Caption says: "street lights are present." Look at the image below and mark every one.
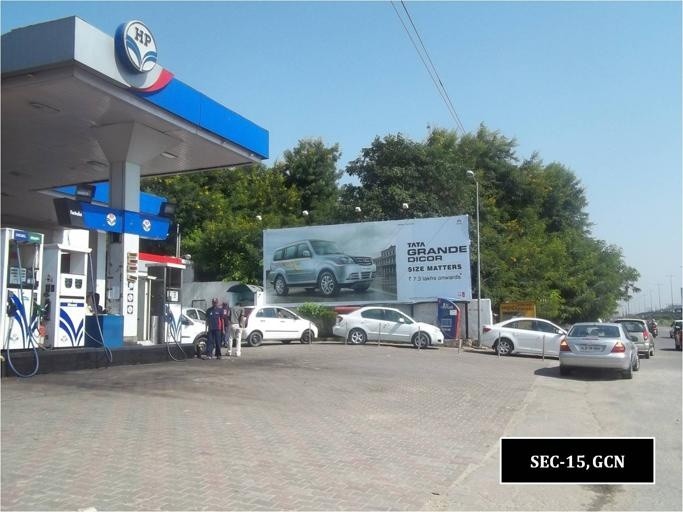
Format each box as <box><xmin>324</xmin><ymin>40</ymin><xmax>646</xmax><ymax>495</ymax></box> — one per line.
<box><xmin>466</xmin><ymin>170</ymin><xmax>481</xmax><ymax>349</ymax></box>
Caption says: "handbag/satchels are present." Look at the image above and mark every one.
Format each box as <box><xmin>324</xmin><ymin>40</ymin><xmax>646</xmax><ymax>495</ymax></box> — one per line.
<box><xmin>238</xmin><ymin>308</ymin><xmax>244</xmax><ymax>328</ymax></box>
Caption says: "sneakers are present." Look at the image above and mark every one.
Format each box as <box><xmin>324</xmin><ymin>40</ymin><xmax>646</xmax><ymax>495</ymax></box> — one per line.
<box><xmin>202</xmin><ymin>351</ymin><xmax>241</xmax><ymax>360</ymax></box>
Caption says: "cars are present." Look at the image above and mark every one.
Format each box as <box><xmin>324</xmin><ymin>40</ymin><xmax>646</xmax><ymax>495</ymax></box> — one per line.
<box><xmin>182</xmin><ymin>307</ymin><xmax>212</xmax><ymax>355</ymax></box>
<box><xmin>670</xmin><ymin>320</ymin><xmax>683</xmax><ymax>351</ymax></box>
<box><xmin>480</xmin><ymin>317</ymin><xmax>654</xmax><ymax>379</ymax></box>
<box><xmin>242</xmin><ymin>305</ymin><xmax>318</xmax><ymax>347</ymax></box>
<box><xmin>332</xmin><ymin>306</ymin><xmax>444</xmax><ymax>349</ymax></box>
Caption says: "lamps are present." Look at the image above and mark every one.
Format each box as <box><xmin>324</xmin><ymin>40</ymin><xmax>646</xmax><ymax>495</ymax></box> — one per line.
<box><xmin>75</xmin><ymin>184</ymin><xmax>96</xmax><ymax>199</ymax></box>
<box><xmin>159</xmin><ymin>202</ymin><xmax>178</xmax><ymax>217</ymax></box>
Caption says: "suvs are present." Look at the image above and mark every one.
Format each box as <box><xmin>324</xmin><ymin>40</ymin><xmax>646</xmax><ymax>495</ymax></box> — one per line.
<box><xmin>268</xmin><ymin>239</ymin><xmax>376</xmax><ymax>297</ymax></box>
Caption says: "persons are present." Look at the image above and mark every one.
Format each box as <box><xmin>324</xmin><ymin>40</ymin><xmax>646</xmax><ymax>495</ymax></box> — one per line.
<box><xmin>202</xmin><ymin>297</ymin><xmax>224</xmax><ymax>361</ymax></box>
<box><xmin>226</xmin><ymin>301</ymin><xmax>246</xmax><ymax>358</ymax></box>
<box><xmin>218</xmin><ymin>301</ymin><xmax>229</xmax><ymax>349</ymax></box>
<box><xmin>648</xmin><ymin>319</ymin><xmax>658</xmax><ymax>336</ymax></box>
